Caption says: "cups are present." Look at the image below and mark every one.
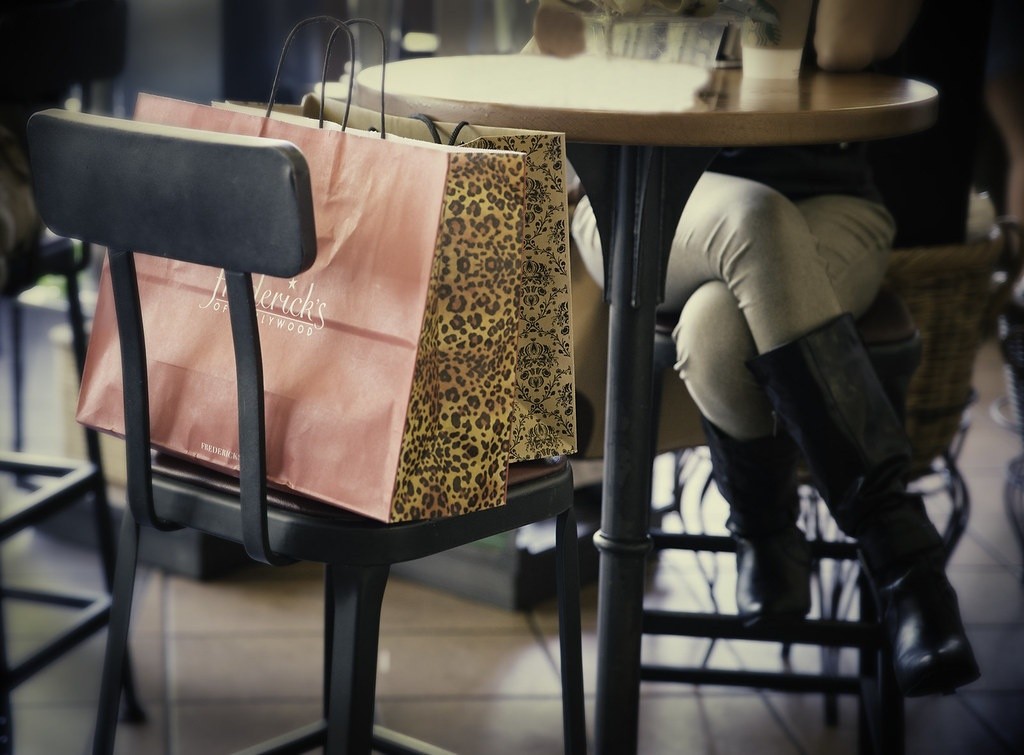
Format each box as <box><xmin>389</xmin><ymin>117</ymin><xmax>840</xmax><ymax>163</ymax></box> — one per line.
<box><xmin>741</xmin><ymin>0</ymin><xmax>811</xmax><ymax>80</ymax></box>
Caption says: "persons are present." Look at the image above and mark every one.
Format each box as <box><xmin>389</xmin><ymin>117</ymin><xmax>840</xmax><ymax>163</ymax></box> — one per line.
<box><xmin>532</xmin><ymin>0</ymin><xmax>986</xmax><ymax>700</ymax></box>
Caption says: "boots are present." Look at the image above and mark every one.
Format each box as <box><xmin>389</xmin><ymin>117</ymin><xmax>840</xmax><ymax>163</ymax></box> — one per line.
<box><xmin>698</xmin><ymin>410</ymin><xmax>822</xmax><ymax>634</ymax></box>
<box><xmin>739</xmin><ymin>307</ymin><xmax>983</xmax><ymax>700</ymax></box>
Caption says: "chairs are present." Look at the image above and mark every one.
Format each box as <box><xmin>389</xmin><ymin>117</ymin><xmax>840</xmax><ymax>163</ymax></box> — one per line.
<box><xmin>552</xmin><ymin>207</ymin><xmax>1011</xmax><ymax>755</ymax></box>
<box><xmin>23</xmin><ymin>107</ymin><xmax>591</xmax><ymax>753</ymax></box>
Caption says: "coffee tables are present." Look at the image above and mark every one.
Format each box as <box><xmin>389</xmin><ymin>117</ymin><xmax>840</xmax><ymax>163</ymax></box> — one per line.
<box><xmin>354</xmin><ymin>44</ymin><xmax>943</xmax><ymax>755</ymax></box>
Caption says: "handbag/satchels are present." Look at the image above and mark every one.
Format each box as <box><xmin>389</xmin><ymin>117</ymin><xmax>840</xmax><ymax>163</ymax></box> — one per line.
<box><xmin>68</xmin><ymin>15</ymin><xmax>579</xmax><ymax>526</ymax></box>
